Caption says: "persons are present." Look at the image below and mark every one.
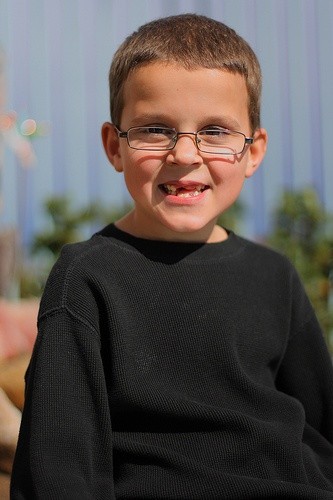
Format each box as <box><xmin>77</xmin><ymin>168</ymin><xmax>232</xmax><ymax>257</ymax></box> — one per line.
<box><xmin>8</xmin><ymin>12</ymin><xmax>333</xmax><ymax>500</ymax></box>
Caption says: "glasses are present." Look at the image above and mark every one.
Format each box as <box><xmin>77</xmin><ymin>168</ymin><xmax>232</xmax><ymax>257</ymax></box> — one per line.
<box><xmin>113</xmin><ymin>123</ymin><xmax>254</xmax><ymax>155</ymax></box>
